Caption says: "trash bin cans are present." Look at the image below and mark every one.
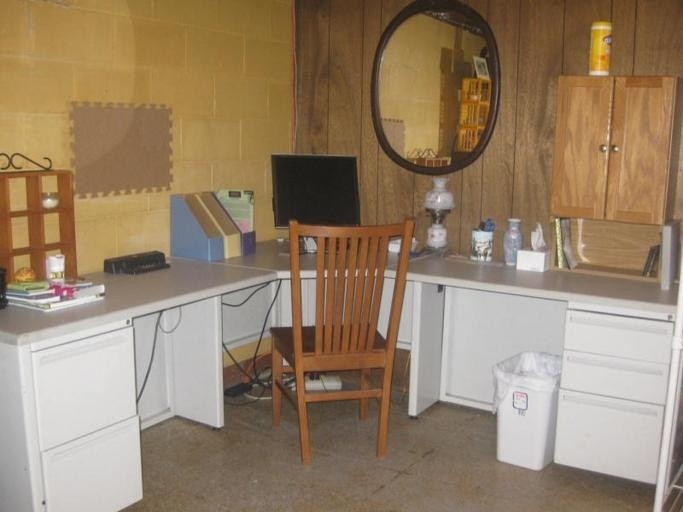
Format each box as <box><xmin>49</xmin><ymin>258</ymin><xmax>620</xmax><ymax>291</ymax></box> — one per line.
<box><xmin>493</xmin><ymin>350</ymin><xmax>562</xmax><ymax>472</ymax></box>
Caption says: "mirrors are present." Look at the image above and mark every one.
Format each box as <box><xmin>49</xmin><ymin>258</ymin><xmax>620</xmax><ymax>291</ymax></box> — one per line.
<box><xmin>370</xmin><ymin>0</ymin><xmax>500</xmax><ymax>178</ymax></box>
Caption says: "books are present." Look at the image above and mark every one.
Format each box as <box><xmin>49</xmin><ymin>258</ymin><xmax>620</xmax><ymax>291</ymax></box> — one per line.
<box><xmin>5</xmin><ymin>275</ymin><xmax>107</xmax><ymax>313</ymax></box>
<box><xmin>553</xmin><ymin>215</ymin><xmax>577</xmax><ymax>270</ymax></box>
<box><xmin>640</xmin><ymin>244</ymin><xmax>660</xmax><ymax>276</ymax></box>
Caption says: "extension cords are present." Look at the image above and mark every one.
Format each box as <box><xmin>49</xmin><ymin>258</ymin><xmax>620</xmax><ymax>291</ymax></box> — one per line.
<box><xmin>302</xmin><ymin>375</ymin><xmax>342</xmax><ymax>390</ymax></box>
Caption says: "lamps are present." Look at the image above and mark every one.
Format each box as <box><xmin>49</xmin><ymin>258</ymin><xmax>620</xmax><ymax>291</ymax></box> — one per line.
<box><xmin>420</xmin><ymin>175</ymin><xmax>458</xmax><ymax>266</ymax></box>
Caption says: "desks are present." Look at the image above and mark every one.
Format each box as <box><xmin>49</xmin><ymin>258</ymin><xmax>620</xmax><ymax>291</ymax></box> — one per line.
<box><xmin>1</xmin><ymin>240</ymin><xmax>682</xmax><ymax>511</ymax></box>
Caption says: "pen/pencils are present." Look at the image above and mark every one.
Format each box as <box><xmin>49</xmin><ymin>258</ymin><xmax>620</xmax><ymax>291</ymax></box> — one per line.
<box><xmin>477</xmin><ymin>217</ymin><xmax>496</xmax><ymax>231</ymax></box>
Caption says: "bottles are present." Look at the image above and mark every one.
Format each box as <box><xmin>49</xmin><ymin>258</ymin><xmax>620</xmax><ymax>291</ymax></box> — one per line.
<box><xmin>589</xmin><ymin>20</ymin><xmax>612</xmax><ymax>76</ymax></box>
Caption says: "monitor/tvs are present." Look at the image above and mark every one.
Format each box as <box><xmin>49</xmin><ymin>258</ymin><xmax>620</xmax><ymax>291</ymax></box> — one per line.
<box><xmin>272</xmin><ymin>155</ymin><xmax>360</xmax><ymax>256</ymax></box>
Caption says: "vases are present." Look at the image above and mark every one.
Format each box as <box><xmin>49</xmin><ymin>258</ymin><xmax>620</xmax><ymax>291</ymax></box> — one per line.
<box><xmin>502</xmin><ymin>216</ymin><xmax>523</xmax><ymax>266</ymax></box>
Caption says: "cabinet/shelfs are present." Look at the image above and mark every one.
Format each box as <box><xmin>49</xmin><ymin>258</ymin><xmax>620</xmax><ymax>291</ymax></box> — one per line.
<box><xmin>552</xmin><ymin>72</ymin><xmax>677</xmax><ymax>226</ymax></box>
<box><xmin>455</xmin><ymin>71</ymin><xmax>490</xmax><ymax>154</ymax></box>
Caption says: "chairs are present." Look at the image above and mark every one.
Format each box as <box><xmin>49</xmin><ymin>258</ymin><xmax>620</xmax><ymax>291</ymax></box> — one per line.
<box><xmin>271</xmin><ymin>215</ymin><xmax>416</xmax><ymax>466</ymax></box>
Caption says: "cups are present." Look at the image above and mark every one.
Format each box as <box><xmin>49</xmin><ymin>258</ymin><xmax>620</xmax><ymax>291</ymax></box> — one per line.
<box><xmin>470</xmin><ymin>228</ymin><xmax>493</xmax><ymax>262</ymax></box>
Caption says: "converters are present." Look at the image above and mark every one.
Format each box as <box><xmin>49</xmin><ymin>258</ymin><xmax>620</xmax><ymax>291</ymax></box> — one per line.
<box><xmin>224</xmin><ymin>383</ymin><xmax>252</xmax><ymax>397</ymax></box>
